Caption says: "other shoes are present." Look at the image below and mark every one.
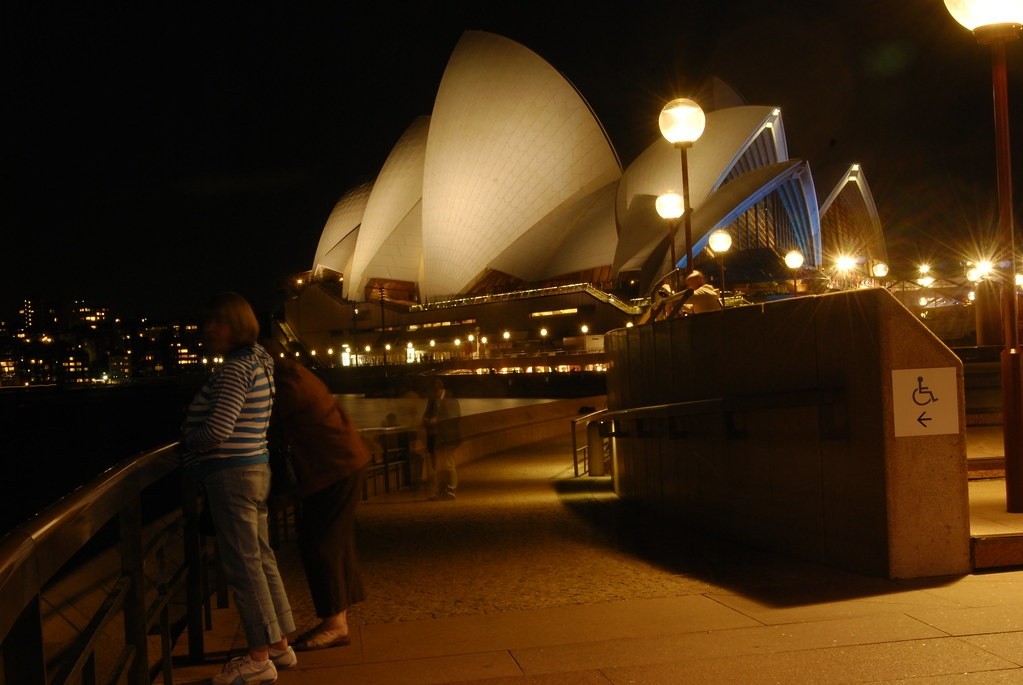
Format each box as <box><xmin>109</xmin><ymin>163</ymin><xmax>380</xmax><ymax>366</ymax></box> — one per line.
<box><xmin>444</xmin><ymin>490</ymin><xmax>456</xmax><ymax>499</ymax></box>
<box><xmin>294</xmin><ymin>622</ymin><xmax>349</xmax><ymax>650</ymax></box>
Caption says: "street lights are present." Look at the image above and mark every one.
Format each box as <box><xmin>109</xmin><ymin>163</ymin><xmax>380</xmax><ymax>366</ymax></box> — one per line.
<box><xmin>945</xmin><ymin>0</ymin><xmax>1023</xmax><ymax>516</ymax></box>
<box><xmin>966</xmin><ymin>267</ymin><xmax>985</xmax><ymax>347</ymax></box>
<box><xmin>660</xmin><ymin>97</ymin><xmax>706</xmax><ymax>285</ymax></box>
<box><xmin>873</xmin><ymin>261</ymin><xmax>889</xmax><ymax>287</ymax></box>
<box><xmin>838</xmin><ymin>255</ymin><xmax>855</xmax><ymax>291</ymax></box>
<box><xmin>709</xmin><ymin>229</ymin><xmax>732</xmax><ymax>308</ymax></box>
<box><xmin>656</xmin><ymin>188</ymin><xmax>684</xmax><ymax>296</ymax></box>
<box><xmin>785</xmin><ymin>250</ymin><xmax>804</xmax><ymax>299</ymax></box>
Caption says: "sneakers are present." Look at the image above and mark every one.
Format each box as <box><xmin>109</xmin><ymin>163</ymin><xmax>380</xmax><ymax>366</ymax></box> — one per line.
<box><xmin>267</xmin><ymin>644</ymin><xmax>297</xmax><ymax>669</ymax></box>
<box><xmin>210</xmin><ymin>652</ymin><xmax>279</xmax><ymax>685</ymax></box>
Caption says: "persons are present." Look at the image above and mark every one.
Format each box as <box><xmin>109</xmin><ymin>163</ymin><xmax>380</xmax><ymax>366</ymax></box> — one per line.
<box><xmin>188</xmin><ymin>295</ymin><xmax>298</xmax><ymax>685</ymax></box>
<box><xmin>658</xmin><ymin>284</ymin><xmax>677</xmax><ymax>319</ymax></box>
<box><xmin>422</xmin><ymin>376</ymin><xmax>461</xmax><ymax>502</ymax></box>
<box><xmin>379</xmin><ymin>413</ymin><xmax>423</xmax><ymax>491</ymax></box>
<box><xmin>265</xmin><ymin>345</ymin><xmax>373</xmax><ymax>650</ymax></box>
<box><xmin>651</xmin><ymin>269</ymin><xmax>723</xmax><ymax>315</ymax></box>
